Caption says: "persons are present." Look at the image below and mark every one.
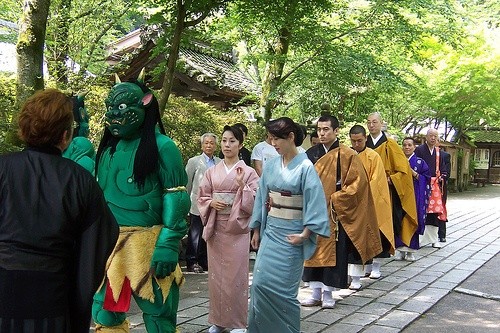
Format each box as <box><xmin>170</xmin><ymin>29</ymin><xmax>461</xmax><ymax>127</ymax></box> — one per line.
<box><xmin>60</xmin><ymin>91</ymin><xmax>97</xmax><ymax>180</ymax></box>
<box><xmin>184</xmin><ymin>111</ymin><xmax>451</xmax><ymax>333</ymax></box>
<box><xmin>0</xmin><ymin>88</ymin><xmax>121</xmax><ymax>333</ymax></box>
<box><xmin>94</xmin><ymin>68</ymin><xmax>190</xmax><ymax>333</ymax></box>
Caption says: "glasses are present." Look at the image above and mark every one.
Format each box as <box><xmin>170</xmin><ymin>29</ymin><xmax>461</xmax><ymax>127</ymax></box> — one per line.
<box><xmin>71</xmin><ymin>120</ymin><xmax>79</xmax><ymax>130</ymax></box>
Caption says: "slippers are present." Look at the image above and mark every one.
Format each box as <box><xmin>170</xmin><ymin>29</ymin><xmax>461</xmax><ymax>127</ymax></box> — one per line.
<box><xmin>299</xmin><ymin>297</ymin><xmax>335</xmax><ymax>309</ymax></box>
<box><xmin>361</xmin><ymin>268</ymin><xmax>382</xmax><ymax>279</ymax></box>
<box><xmin>432</xmin><ymin>242</ymin><xmax>441</xmax><ymax>248</ymax></box>
<box><xmin>349</xmin><ymin>280</ymin><xmax>363</xmax><ymax>291</ymax></box>
<box><xmin>395</xmin><ymin>251</ymin><xmax>416</xmax><ymax>262</ymax></box>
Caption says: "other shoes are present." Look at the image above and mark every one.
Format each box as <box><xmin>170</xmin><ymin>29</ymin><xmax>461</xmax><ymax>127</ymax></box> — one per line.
<box><xmin>187</xmin><ymin>262</ymin><xmax>204</xmax><ymax>273</ymax></box>
<box><xmin>440</xmin><ymin>237</ymin><xmax>446</xmax><ymax>242</ymax></box>
